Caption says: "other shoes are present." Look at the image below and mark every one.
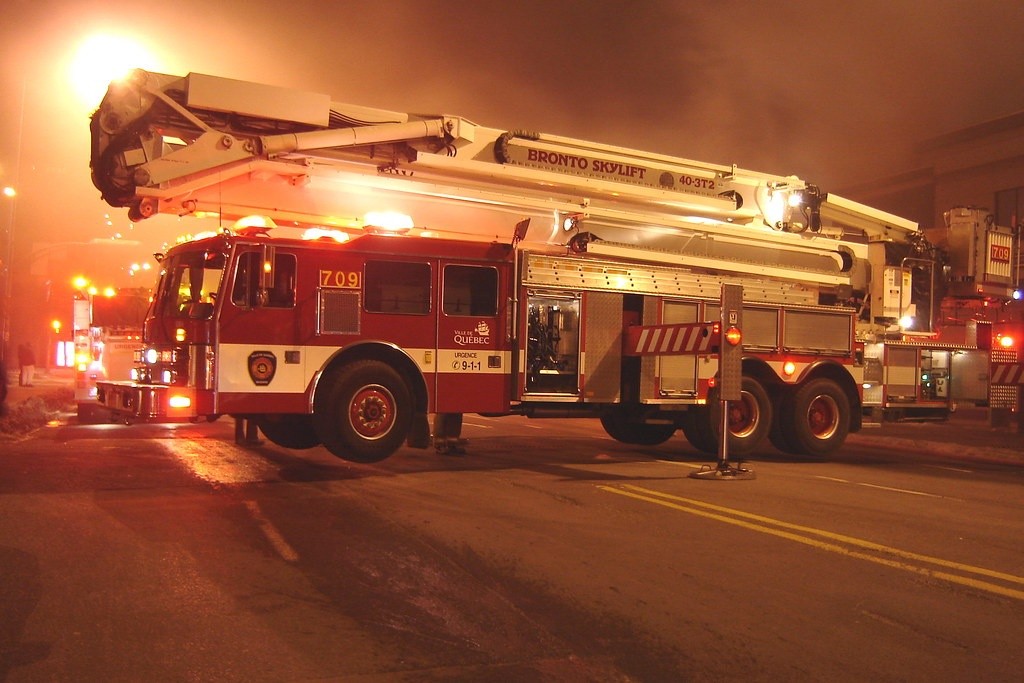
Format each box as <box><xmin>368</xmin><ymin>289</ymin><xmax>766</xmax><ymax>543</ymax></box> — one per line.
<box><xmin>448</xmin><ymin>437</ymin><xmax>469</xmax><ymax>445</ymax></box>
<box><xmin>236</xmin><ymin>439</ymin><xmax>248</xmax><ymax>446</ymax></box>
<box><xmin>248</xmin><ymin>439</ymin><xmax>264</xmax><ymax>445</ymax></box>
<box><xmin>434</xmin><ymin>436</ymin><xmax>447</xmax><ymax>449</ymax></box>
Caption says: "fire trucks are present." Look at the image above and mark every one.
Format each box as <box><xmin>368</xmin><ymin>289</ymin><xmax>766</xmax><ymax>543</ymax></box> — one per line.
<box><xmin>73</xmin><ymin>67</ymin><xmax>1024</xmax><ymax>463</ymax></box>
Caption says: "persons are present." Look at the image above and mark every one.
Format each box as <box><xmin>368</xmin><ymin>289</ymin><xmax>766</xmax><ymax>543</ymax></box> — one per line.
<box><xmin>18</xmin><ymin>342</ymin><xmax>37</xmax><ymax>387</ymax></box>
<box><xmin>431</xmin><ymin>412</ymin><xmax>467</xmax><ymax>454</ymax></box>
